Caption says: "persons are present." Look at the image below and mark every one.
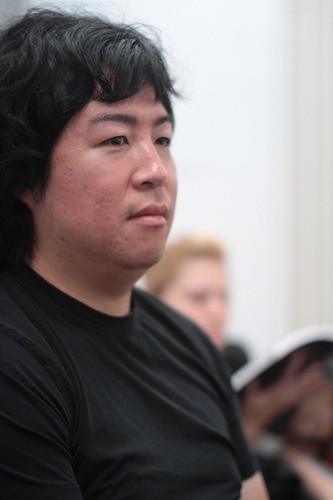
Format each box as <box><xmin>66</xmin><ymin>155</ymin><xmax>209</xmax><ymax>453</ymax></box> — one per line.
<box><xmin>0</xmin><ymin>3</ymin><xmax>277</xmax><ymax>497</ymax></box>
<box><xmin>136</xmin><ymin>230</ymin><xmax>332</xmax><ymax>500</ymax></box>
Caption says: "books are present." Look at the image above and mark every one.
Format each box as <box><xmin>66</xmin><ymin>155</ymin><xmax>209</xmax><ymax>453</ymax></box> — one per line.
<box><xmin>228</xmin><ymin>327</ymin><xmax>332</xmax><ymax>396</ymax></box>
